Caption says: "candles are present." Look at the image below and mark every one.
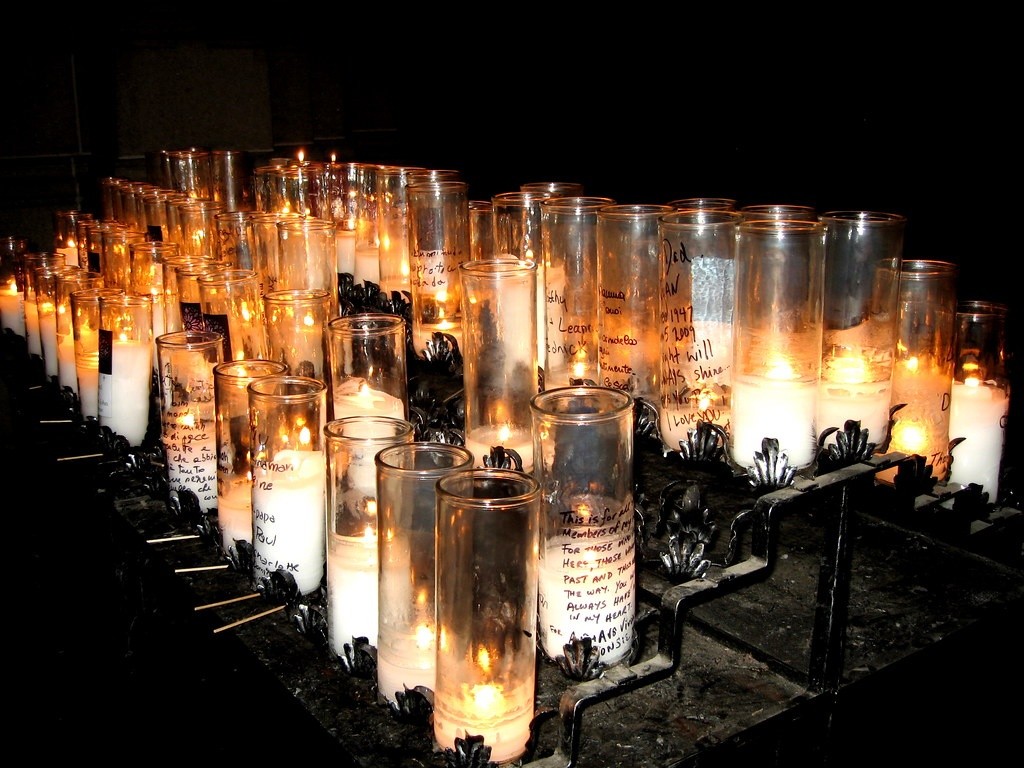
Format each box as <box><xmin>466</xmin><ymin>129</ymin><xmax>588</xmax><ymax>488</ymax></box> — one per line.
<box><xmin>0</xmin><ymin>148</ymin><xmax>1014</xmax><ymax>768</ymax></box>
